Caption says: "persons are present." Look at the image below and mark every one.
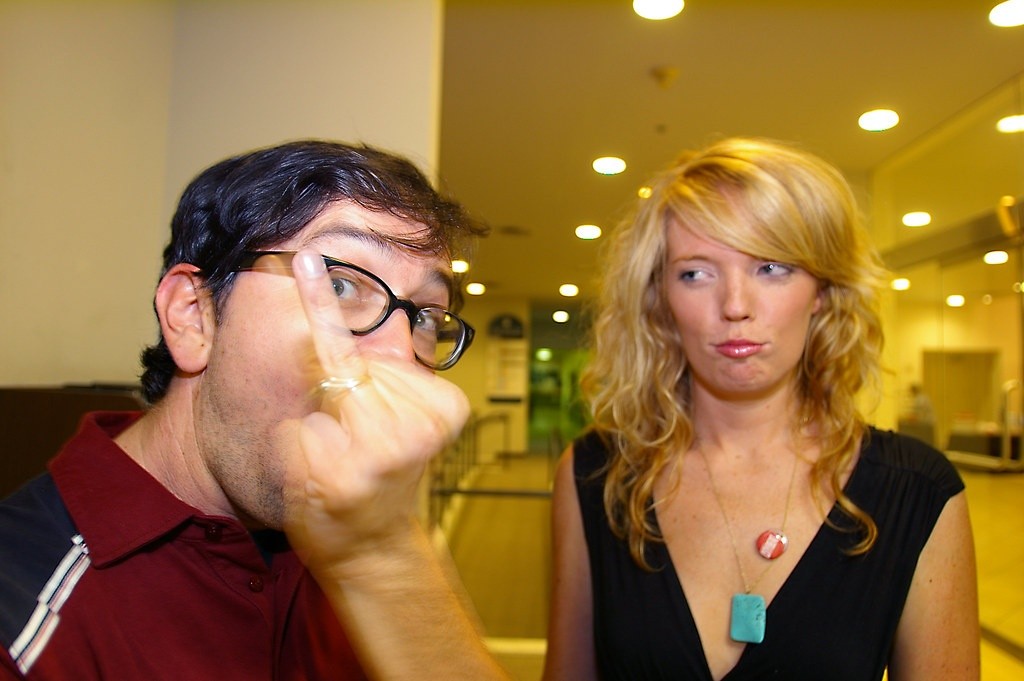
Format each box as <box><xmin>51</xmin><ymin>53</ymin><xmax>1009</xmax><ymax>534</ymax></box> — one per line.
<box><xmin>542</xmin><ymin>136</ymin><xmax>981</xmax><ymax>681</ymax></box>
<box><xmin>0</xmin><ymin>138</ymin><xmax>511</xmax><ymax>681</ymax></box>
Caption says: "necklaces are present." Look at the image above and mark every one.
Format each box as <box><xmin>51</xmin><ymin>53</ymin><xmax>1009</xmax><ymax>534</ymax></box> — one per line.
<box><xmin>695</xmin><ymin>422</ymin><xmax>801</xmax><ymax>644</ymax></box>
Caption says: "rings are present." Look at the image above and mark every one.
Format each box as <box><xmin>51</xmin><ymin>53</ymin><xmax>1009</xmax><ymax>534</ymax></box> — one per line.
<box><xmin>319</xmin><ymin>374</ymin><xmax>372</xmax><ymax>401</ymax></box>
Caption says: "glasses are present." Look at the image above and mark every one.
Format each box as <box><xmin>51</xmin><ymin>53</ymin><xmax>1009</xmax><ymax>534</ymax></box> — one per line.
<box><xmin>235</xmin><ymin>250</ymin><xmax>476</xmax><ymax>370</ymax></box>
